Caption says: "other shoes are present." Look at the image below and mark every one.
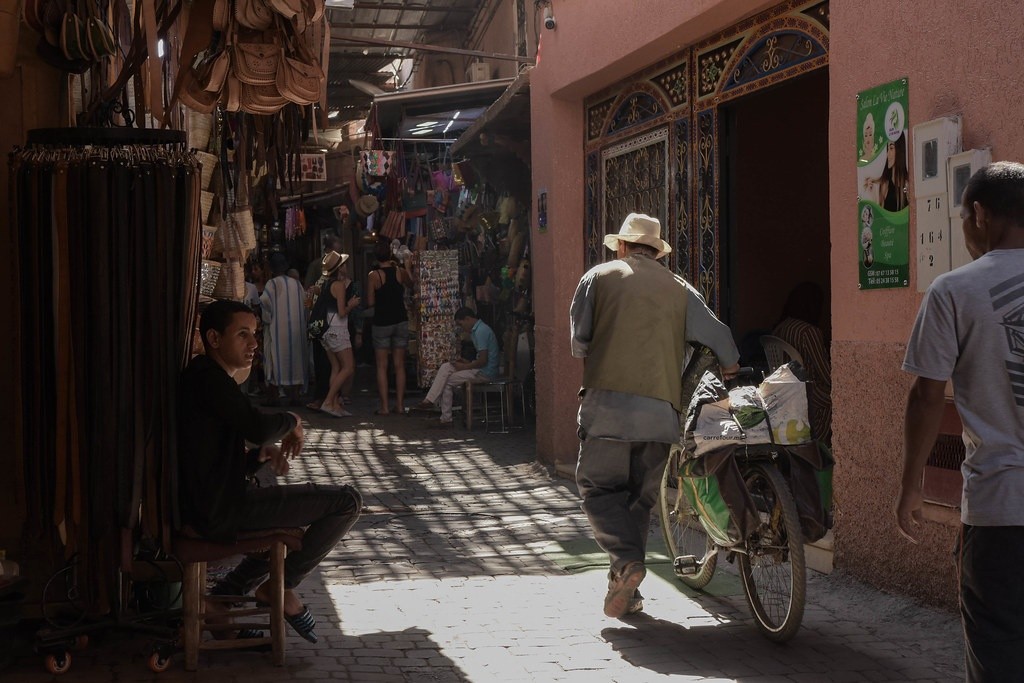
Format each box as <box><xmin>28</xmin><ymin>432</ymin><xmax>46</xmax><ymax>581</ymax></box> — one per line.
<box><xmin>412</xmin><ymin>402</ymin><xmax>433</xmax><ymax>409</ymax></box>
<box><xmin>343</xmin><ymin>410</ymin><xmax>352</xmax><ymax>416</ymax></box>
<box><xmin>427</xmin><ymin>420</ymin><xmax>453</xmax><ymax>430</ymax></box>
<box><xmin>604</xmin><ymin>560</ymin><xmax>646</xmax><ymax>618</ymax></box>
<box><xmin>320</xmin><ymin>404</ymin><xmax>342</xmax><ymax>417</ymax></box>
<box><xmin>305</xmin><ymin>402</ymin><xmax>321</xmax><ymax>410</ymax></box>
<box><xmin>343</xmin><ymin>394</ymin><xmax>352</xmax><ymax>404</ymax></box>
<box><xmin>627</xmin><ymin>600</ymin><xmax>643</xmax><ymax>614</ymax></box>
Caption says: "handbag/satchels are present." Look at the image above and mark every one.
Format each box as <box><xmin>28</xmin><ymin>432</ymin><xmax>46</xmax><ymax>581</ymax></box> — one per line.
<box><xmin>178</xmin><ymin>0</ymin><xmax>326</xmax><ymax>115</ymax></box>
<box><xmin>212</xmin><ymin>261</ymin><xmax>245</xmax><ymax>299</ymax></box>
<box><xmin>210</xmin><ymin>210</ymin><xmax>256</xmax><ymax>252</ymax></box>
<box><xmin>460</xmin><ymin>240</ymin><xmax>489</xmax><ymax>286</ymax></box>
<box><xmin>356</xmin><ymin>123</ymin><xmax>494</xmax><ymax>245</ymax></box>
<box><xmin>22</xmin><ymin>0</ymin><xmax>117</xmax><ymax>62</ymax></box>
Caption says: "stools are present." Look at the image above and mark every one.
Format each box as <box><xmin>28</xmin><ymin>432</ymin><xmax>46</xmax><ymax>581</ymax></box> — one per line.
<box><xmin>484</xmin><ymin>378</ymin><xmax>528</xmax><ymax>434</ymax></box>
<box><xmin>177</xmin><ymin>526</ymin><xmax>304</xmax><ymax>673</ymax></box>
<box><xmin>464</xmin><ymin>380</ymin><xmax>514</xmax><ymax>432</ymax></box>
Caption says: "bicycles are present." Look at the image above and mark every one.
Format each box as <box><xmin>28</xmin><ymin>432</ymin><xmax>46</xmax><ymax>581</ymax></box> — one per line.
<box><xmin>657</xmin><ymin>366</ymin><xmax>807</xmax><ymax>645</ymax></box>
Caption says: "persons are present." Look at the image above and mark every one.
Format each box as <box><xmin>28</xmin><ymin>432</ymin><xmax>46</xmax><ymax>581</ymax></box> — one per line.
<box><xmin>180</xmin><ymin>297</ymin><xmax>363</xmax><ymax>645</ymax></box>
<box><xmin>259</xmin><ymin>233</ymin><xmax>504</xmax><ymax>428</ymax></box>
<box><xmin>861</xmin><ymin>131</ymin><xmax>912</xmax><ymax>213</ymax></box>
<box><xmin>568</xmin><ymin>213</ymin><xmax>739</xmax><ymax>617</ymax></box>
<box><xmin>895</xmin><ymin>162</ymin><xmax>1024</xmax><ymax>683</ymax></box>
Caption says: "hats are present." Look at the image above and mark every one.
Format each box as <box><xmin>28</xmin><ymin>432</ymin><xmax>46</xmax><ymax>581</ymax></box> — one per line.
<box><xmin>360</xmin><ymin>194</ymin><xmax>379</xmax><ymax>214</ymax></box>
<box><xmin>322</xmin><ymin>250</ymin><xmax>350</xmax><ymax>275</ymax></box>
<box><xmin>604</xmin><ymin>212</ymin><xmax>672</xmax><ymax>260</ymax></box>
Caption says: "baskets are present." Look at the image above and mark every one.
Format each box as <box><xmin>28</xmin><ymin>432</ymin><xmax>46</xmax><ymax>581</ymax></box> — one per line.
<box><xmin>183</xmin><ymin>105</ymin><xmax>222</xmax><ymax>315</ymax></box>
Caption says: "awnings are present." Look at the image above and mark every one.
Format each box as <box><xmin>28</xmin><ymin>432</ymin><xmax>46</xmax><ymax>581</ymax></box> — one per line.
<box><xmin>362</xmin><ymin>77</ymin><xmax>515</xmax><ymax>139</ymax></box>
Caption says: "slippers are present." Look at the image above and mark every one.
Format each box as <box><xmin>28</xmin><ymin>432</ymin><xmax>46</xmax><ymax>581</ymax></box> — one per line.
<box><xmin>374</xmin><ymin>410</ymin><xmax>389</xmax><ymax>417</ymax></box>
<box><xmin>256</xmin><ymin>601</ymin><xmax>318</xmax><ymax>643</ymax></box>
<box><xmin>391</xmin><ymin>408</ymin><xmax>404</xmax><ymax>415</ymax></box>
<box><xmin>236</xmin><ymin>629</ymin><xmax>272</xmax><ymax>652</ymax></box>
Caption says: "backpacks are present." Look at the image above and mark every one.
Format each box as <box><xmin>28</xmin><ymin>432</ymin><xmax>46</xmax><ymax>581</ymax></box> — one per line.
<box><xmin>307</xmin><ymin>279</ymin><xmax>339</xmax><ymax>340</ymax></box>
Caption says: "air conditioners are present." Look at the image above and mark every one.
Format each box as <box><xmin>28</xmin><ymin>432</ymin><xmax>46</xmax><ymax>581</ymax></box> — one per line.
<box><xmin>465</xmin><ymin>63</ymin><xmax>490</xmax><ymax>82</ymax></box>
